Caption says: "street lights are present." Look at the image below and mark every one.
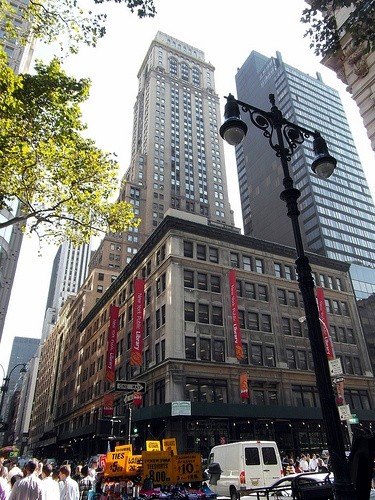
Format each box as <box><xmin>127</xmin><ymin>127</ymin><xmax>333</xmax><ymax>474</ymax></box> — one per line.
<box><xmin>0</xmin><ymin>363</ymin><xmax>27</xmax><ymax>416</ymax></box>
<box><xmin>219</xmin><ymin>93</ymin><xmax>356</xmax><ymax>500</ymax></box>
<box><xmin>299</xmin><ymin>316</ymin><xmax>353</xmax><ymax>446</ymax></box>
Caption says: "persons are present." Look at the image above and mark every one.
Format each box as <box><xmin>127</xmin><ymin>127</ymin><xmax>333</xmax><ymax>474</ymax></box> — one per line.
<box><xmin>91</xmin><ymin>460</ymin><xmax>104</xmax><ymax>493</ymax></box>
<box><xmin>0</xmin><ymin>457</ymin><xmax>93</xmax><ymax>484</ymax></box>
<box><xmin>279</xmin><ymin>449</ymin><xmax>326</xmax><ymax>476</ymax></box>
<box><xmin>8</xmin><ymin>474</ymin><xmax>23</xmax><ymax>500</ymax></box>
<box><xmin>7</xmin><ymin>461</ymin><xmax>43</xmax><ymax>500</ymax></box>
<box><xmin>77</xmin><ymin>466</ymin><xmax>94</xmax><ymax>500</ymax></box>
<box><xmin>39</xmin><ymin>464</ymin><xmax>61</xmax><ymax>500</ymax></box>
<box><xmin>72</xmin><ymin>465</ymin><xmax>86</xmax><ymax>482</ymax></box>
<box><xmin>0</xmin><ymin>466</ymin><xmax>8</xmax><ymax>500</ymax></box>
<box><xmin>219</xmin><ymin>436</ymin><xmax>226</xmax><ymax>445</ymax></box>
<box><xmin>57</xmin><ymin>465</ymin><xmax>79</xmax><ymax>500</ymax></box>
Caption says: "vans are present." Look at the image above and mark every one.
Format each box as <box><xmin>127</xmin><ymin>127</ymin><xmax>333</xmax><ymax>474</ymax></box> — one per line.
<box><xmin>204</xmin><ymin>441</ymin><xmax>284</xmax><ymax>500</ymax></box>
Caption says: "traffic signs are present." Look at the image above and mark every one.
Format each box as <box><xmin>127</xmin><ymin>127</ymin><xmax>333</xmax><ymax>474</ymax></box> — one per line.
<box><xmin>115</xmin><ymin>380</ymin><xmax>146</xmax><ymax>393</ymax></box>
<box><xmin>331</xmin><ymin>377</ymin><xmax>344</xmax><ymax>384</ymax></box>
<box><xmin>122</xmin><ymin>393</ymin><xmax>134</xmax><ymax>406</ymax></box>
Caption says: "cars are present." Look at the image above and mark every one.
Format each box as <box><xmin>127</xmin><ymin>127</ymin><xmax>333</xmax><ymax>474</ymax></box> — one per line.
<box><xmin>264</xmin><ymin>472</ymin><xmax>375</xmax><ymax>500</ymax></box>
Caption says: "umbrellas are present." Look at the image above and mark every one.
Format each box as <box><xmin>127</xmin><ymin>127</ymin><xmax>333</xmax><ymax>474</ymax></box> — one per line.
<box><xmin>0</xmin><ymin>445</ymin><xmax>20</xmax><ymax>451</ymax></box>
